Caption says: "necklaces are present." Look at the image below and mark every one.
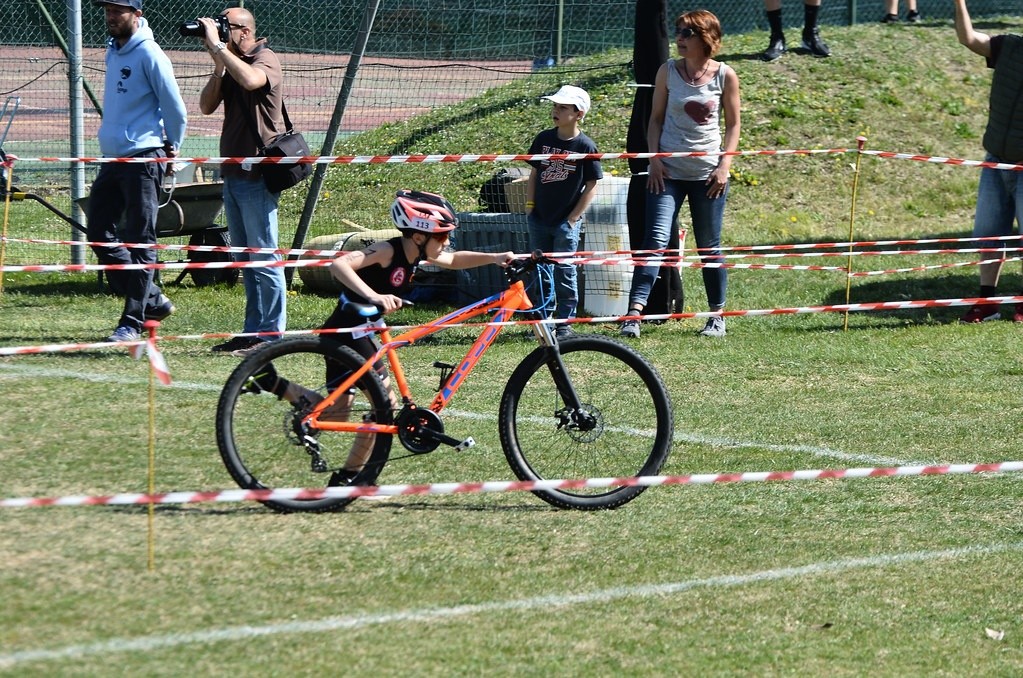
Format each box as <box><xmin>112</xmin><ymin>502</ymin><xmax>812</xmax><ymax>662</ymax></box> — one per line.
<box><xmin>684</xmin><ymin>58</ymin><xmax>710</xmax><ymax>85</ymax></box>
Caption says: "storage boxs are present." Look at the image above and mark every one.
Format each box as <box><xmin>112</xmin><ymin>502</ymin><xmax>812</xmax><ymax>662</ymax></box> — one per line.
<box><xmin>454</xmin><ymin>172</ymin><xmax>687</xmax><ymax>316</ymax></box>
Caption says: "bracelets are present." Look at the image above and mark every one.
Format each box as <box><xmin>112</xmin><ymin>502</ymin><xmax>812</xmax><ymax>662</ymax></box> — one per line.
<box><xmin>213</xmin><ymin>73</ymin><xmax>222</xmax><ymax>80</ymax></box>
<box><xmin>526</xmin><ymin>201</ymin><xmax>535</xmax><ymax>208</ymax></box>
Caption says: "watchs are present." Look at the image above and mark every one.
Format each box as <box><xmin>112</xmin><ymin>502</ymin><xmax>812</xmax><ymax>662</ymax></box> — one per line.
<box><xmin>212</xmin><ymin>41</ymin><xmax>226</xmax><ymax>55</ymax></box>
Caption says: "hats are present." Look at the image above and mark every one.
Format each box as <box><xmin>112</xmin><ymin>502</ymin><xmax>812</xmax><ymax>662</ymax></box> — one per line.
<box><xmin>541</xmin><ymin>85</ymin><xmax>591</xmax><ymax>119</ymax></box>
<box><xmin>93</xmin><ymin>0</ymin><xmax>141</xmax><ymax>10</ymax></box>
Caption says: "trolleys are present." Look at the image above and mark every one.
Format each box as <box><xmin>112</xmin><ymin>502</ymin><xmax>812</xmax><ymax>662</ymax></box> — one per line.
<box><xmin>11</xmin><ymin>180</ymin><xmax>241</xmax><ymax>288</ymax></box>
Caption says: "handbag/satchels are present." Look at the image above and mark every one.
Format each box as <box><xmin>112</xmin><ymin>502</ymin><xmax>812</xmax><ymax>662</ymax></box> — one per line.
<box><xmin>502</xmin><ymin>167</ymin><xmax>531</xmax><ymax>177</ymax></box>
<box><xmin>478</xmin><ymin>173</ymin><xmax>510</xmax><ymax>212</ymax></box>
<box><xmin>256</xmin><ymin>134</ymin><xmax>313</xmax><ymax>192</ymax></box>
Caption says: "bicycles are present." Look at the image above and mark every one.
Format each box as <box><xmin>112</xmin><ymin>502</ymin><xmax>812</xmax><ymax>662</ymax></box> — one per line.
<box><xmin>215</xmin><ymin>249</ymin><xmax>675</xmax><ymax>512</ymax></box>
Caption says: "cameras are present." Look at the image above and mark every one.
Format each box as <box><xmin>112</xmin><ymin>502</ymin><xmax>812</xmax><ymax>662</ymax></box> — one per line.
<box><xmin>178</xmin><ymin>15</ymin><xmax>230</xmax><ymax>42</ymax></box>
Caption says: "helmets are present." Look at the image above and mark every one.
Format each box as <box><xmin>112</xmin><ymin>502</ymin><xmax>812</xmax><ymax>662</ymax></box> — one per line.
<box><xmin>390</xmin><ymin>190</ymin><xmax>459</xmax><ymax>233</ymax></box>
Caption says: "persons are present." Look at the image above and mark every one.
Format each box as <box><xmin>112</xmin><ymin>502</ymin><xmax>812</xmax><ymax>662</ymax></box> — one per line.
<box><xmin>619</xmin><ymin>9</ymin><xmax>741</xmax><ymax>337</ymax></box>
<box><xmin>522</xmin><ymin>84</ymin><xmax>602</xmax><ymax>339</ymax></box>
<box><xmin>196</xmin><ymin>8</ymin><xmax>287</xmax><ymax>354</ymax></box>
<box><xmin>955</xmin><ymin>0</ymin><xmax>1023</xmax><ymax>325</ymax></box>
<box><xmin>237</xmin><ymin>188</ymin><xmax>519</xmax><ymax>496</ymax></box>
<box><xmin>761</xmin><ymin>0</ymin><xmax>832</xmax><ymax>61</ymax></box>
<box><xmin>880</xmin><ymin>0</ymin><xmax>921</xmax><ymax>23</ymax></box>
<box><xmin>87</xmin><ymin>0</ymin><xmax>187</xmax><ymax>342</ymax></box>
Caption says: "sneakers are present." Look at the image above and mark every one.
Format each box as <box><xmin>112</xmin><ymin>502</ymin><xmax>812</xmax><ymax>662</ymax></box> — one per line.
<box><xmin>107</xmin><ymin>323</ymin><xmax>142</xmax><ymax>342</ymax></box>
<box><xmin>211</xmin><ymin>332</ymin><xmax>271</xmax><ymax>354</ymax></box>
<box><xmin>327</xmin><ymin>473</ymin><xmax>377</xmax><ymax>487</ymax></box>
<box><xmin>880</xmin><ymin>13</ymin><xmax>898</xmax><ymax>24</ymax></box>
<box><xmin>523</xmin><ymin>323</ymin><xmax>556</xmax><ymax>338</ymax></box>
<box><xmin>801</xmin><ymin>28</ymin><xmax>832</xmax><ymax>56</ymax></box>
<box><xmin>1013</xmin><ymin>303</ymin><xmax>1023</xmax><ymax>322</ymax></box>
<box><xmin>143</xmin><ymin>293</ymin><xmax>176</xmax><ymax>330</ymax></box>
<box><xmin>907</xmin><ymin>10</ymin><xmax>921</xmax><ymax>23</ymax></box>
<box><xmin>762</xmin><ymin>34</ymin><xmax>787</xmax><ymax>61</ymax></box>
<box><xmin>959</xmin><ymin>304</ymin><xmax>1001</xmax><ymax>324</ymax></box>
<box><xmin>556</xmin><ymin>324</ymin><xmax>573</xmax><ymax>339</ymax></box>
<box><xmin>700</xmin><ymin>316</ymin><xmax>726</xmax><ymax>337</ymax></box>
<box><xmin>617</xmin><ymin>314</ymin><xmax>641</xmax><ymax>338</ymax></box>
<box><xmin>238</xmin><ymin>360</ymin><xmax>277</xmax><ymax>395</ymax></box>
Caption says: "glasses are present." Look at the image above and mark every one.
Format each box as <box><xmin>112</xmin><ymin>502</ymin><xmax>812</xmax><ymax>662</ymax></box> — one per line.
<box><xmin>422</xmin><ymin>231</ymin><xmax>449</xmax><ymax>243</ymax></box>
<box><xmin>674</xmin><ymin>26</ymin><xmax>698</xmax><ymax>40</ymax></box>
